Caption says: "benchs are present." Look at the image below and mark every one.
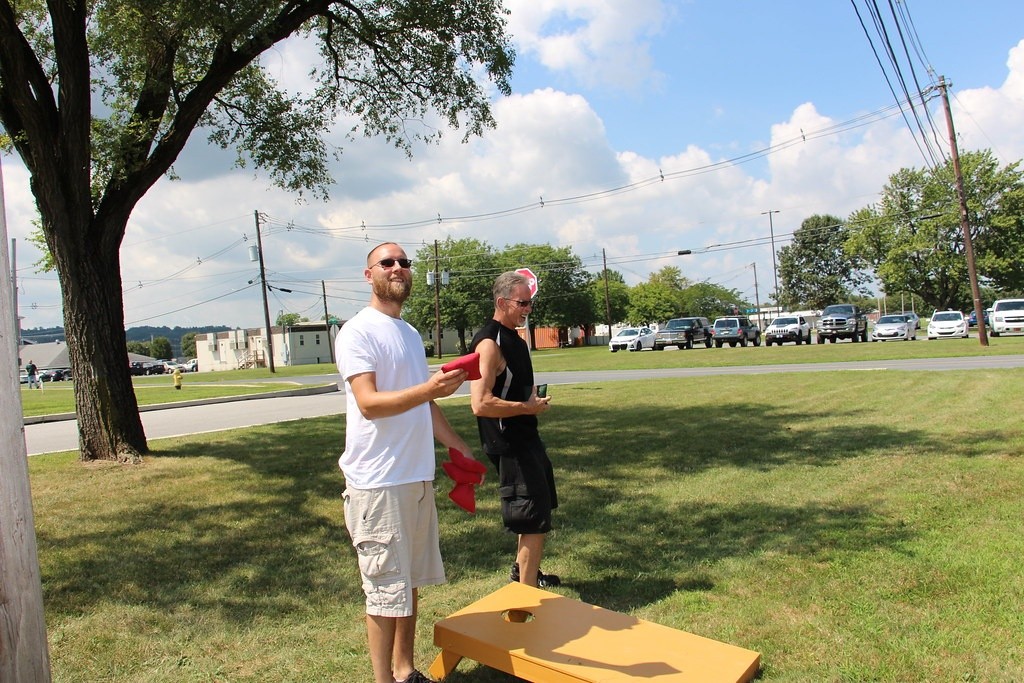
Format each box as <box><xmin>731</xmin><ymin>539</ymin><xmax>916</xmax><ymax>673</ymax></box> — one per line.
<box><xmin>425</xmin><ymin>577</ymin><xmax>761</xmax><ymax>682</ymax></box>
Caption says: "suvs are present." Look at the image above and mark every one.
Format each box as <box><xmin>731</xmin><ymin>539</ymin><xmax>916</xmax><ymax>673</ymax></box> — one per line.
<box><xmin>654</xmin><ymin>317</ymin><xmax>712</xmax><ymax>350</ymax></box>
<box><xmin>711</xmin><ymin>317</ymin><xmax>762</xmax><ymax>348</ymax></box>
<box><xmin>765</xmin><ymin>316</ymin><xmax>812</xmax><ymax>346</ymax></box>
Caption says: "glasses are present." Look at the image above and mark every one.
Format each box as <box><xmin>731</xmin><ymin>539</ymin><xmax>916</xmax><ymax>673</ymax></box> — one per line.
<box><xmin>368</xmin><ymin>258</ymin><xmax>412</xmax><ymax>269</ymax></box>
<box><xmin>502</xmin><ymin>297</ymin><xmax>533</xmax><ymax>307</ymax></box>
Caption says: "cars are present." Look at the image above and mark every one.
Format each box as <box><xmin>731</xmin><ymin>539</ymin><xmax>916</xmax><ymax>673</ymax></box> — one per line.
<box><xmin>19</xmin><ymin>367</ymin><xmax>71</xmax><ymax>383</ymax></box>
<box><xmin>967</xmin><ymin>311</ymin><xmax>989</xmax><ymax>327</ymax></box>
<box><xmin>129</xmin><ymin>361</ymin><xmax>164</xmax><ymax>375</ymax></box>
<box><xmin>192</xmin><ymin>361</ymin><xmax>198</xmax><ymax>372</ymax></box>
<box><xmin>164</xmin><ymin>361</ymin><xmax>185</xmax><ymax>374</ymax></box>
<box><xmin>872</xmin><ymin>315</ymin><xmax>917</xmax><ymax>343</ymax></box>
<box><xmin>986</xmin><ymin>300</ymin><xmax>1024</xmax><ymax>337</ymax></box>
<box><xmin>905</xmin><ymin>312</ymin><xmax>921</xmax><ymax>329</ymax></box>
<box><xmin>926</xmin><ymin>312</ymin><xmax>969</xmax><ymax>339</ymax></box>
<box><xmin>609</xmin><ymin>328</ymin><xmax>656</xmax><ymax>353</ymax></box>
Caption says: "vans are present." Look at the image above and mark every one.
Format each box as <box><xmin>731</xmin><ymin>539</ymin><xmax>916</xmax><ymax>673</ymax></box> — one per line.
<box><xmin>184</xmin><ymin>359</ymin><xmax>197</xmax><ymax>371</ymax></box>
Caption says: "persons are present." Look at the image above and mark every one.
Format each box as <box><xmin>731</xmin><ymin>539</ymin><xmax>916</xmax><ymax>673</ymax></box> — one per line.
<box><xmin>25</xmin><ymin>360</ymin><xmax>40</xmax><ymax>389</ymax></box>
<box><xmin>335</xmin><ymin>242</ymin><xmax>484</xmax><ymax>683</ymax></box>
<box><xmin>468</xmin><ymin>271</ymin><xmax>562</xmax><ymax>623</ymax></box>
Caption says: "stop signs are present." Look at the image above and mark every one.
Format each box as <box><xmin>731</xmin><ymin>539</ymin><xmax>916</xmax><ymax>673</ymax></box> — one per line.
<box><xmin>516</xmin><ymin>268</ymin><xmax>538</xmax><ymax>300</ymax></box>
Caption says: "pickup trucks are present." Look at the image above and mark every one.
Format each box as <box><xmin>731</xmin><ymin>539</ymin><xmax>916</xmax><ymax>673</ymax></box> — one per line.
<box><xmin>816</xmin><ymin>305</ymin><xmax>868</xmax><ymax>344</ymax></box>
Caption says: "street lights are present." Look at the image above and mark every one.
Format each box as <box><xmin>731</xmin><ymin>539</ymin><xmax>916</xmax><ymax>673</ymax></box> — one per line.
<box><xmin>760</xmin><ymin>210</ymin><xmax>781</xmax><ymax>316</ymax></box>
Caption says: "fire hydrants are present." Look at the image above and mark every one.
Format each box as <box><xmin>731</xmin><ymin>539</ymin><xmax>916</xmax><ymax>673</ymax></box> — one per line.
<box><xmin>172</xmin><ymin>368</ymin><xmax>183</xmax><ymax>389</ymax></box>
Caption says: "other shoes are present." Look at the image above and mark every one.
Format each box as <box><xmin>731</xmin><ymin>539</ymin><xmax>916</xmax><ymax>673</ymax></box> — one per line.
<box><xmin>37</xmin><ymin>386</ymin><xmax>39</xmax><ymax>389</ymax></box>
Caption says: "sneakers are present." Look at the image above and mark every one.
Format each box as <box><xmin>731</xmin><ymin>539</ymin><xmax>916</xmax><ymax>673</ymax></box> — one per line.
<box><xmin>391</xmin><ymin>669</ymin><xmax>436</xmax><ymax>683</ymax></box>
<box><xmin>510</xmin><ymin>563</ymin><xmax>560</xmax><ymax>590</ymax></box>
